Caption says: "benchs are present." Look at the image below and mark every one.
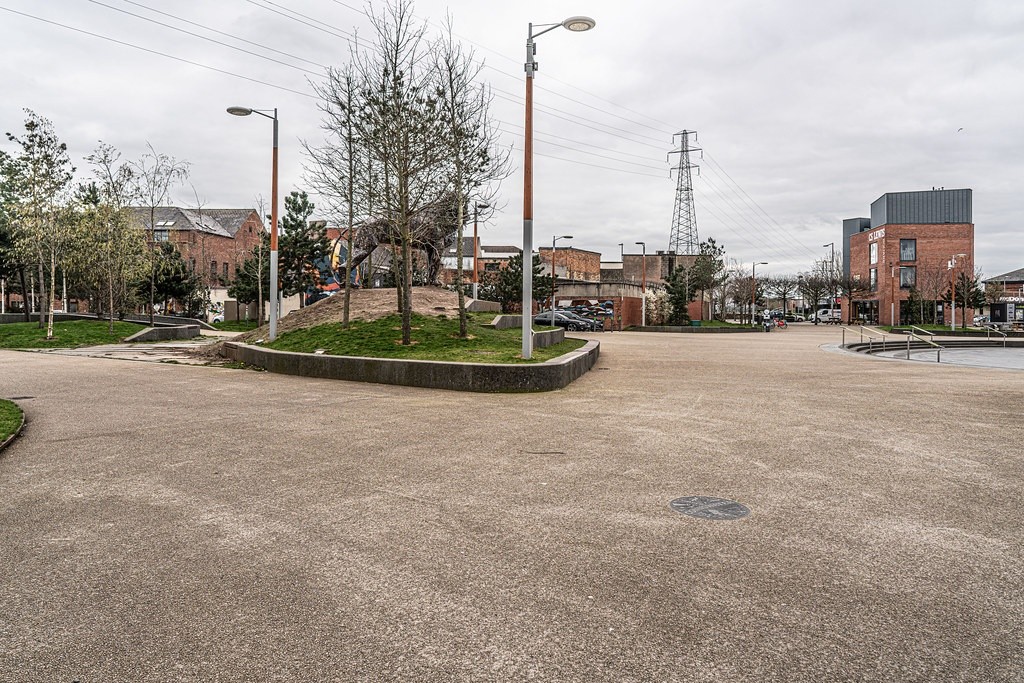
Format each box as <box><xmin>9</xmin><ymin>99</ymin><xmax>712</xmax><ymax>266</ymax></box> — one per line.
<box><xmin>1012</xmin><ymin>326</ymin><xmax>1024</xmax><ymax>331</ymax></box>
<box><xmin>823</xmin><ymin>320</ymin><xmax>843</xmax><ymax>325</ymax></box>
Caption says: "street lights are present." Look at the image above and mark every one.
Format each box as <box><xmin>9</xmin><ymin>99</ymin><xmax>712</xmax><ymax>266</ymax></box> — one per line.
<box><xmin>721</xmin><ymin>270</ymin><xmax>736</xmax><ymax>317</ymax></box>
<box><xmin>519</xmin><ymin>14</ymin><xmax>597</xmax><ymax>358</ymax></box>
<box><xmin>823</xmin><ymin>243</ymin><xmax>834</xmax><ymax>317</ymax></box>
<box><xmin>472</xmin><ymin>200</ymin><xmax>490</xmax><ymax>300</ymax></box>
<box><xmin>551</xmin><ymin>236</ymin><xmax>574</xmax><ymax>328</ymax></box>
<box><xmin>1003</xmin><ymin>275</ymin><xmax>1009</xmax><ymax>293</ymax></box>
<box><xmin>951</xmin><ymin>253</ymin><xmax>967</xmax><ymax>330</ymax></box>
<box><xmin>635</xmin><ymin>242</ymin><xmax>646</xmax><ymax>327</ymax></box>
<box><xmin>618</xmin><ymin>243</ymin><xmax>623</xmax><ymax>255</ymax></box>
<box><xmin>751</xmin><ymin>261</ymin><xmax>769</xmax><ymax>327</ymax></box>
<box><xmin>226</xmin><ymin>104</ymin><xmax>281</xmax><ymax>341</ymax></box>
<box><xmin>797</xmin><ymin>276</ymin><xmax>803</xmax><ymax>314</ymax></box>
<box><xmin>890</xmin><ymin>266</ymin><xmax>907</xmax><ymax>326</ymax></box>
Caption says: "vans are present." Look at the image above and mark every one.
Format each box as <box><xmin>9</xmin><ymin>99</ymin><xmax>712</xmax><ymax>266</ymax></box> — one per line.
<box><xmin>807</xmin><ymin>308</ymin><xmax>842</xmax><ymax>323</ymax></box>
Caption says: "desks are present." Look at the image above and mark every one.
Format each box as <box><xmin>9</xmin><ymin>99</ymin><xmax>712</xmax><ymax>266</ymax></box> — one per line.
<box><xmin>1013</xmin><ymin>323</ymin><xmax>1024</xmax><ymax>331</ymax></box>
<box><xmin>827</xmin><ymin>317</ymin><xmax>841</xmax><ymax>325</ymax></box>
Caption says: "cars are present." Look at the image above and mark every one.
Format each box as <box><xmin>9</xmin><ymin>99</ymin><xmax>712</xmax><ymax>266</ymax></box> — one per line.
<box><xmin>212</xmin><ymin>309</ymin><xmax>224</xmax><ymax>324</ymax></box>
<box><xmin>770</xmin><ymin>307</ymin><xmax>806</xmax><ymax>322</ymax></box>
<box><xmin>534</xmin><ymin>308</ymin><xmax>604</xmax><ymax>333</ymax></box>
